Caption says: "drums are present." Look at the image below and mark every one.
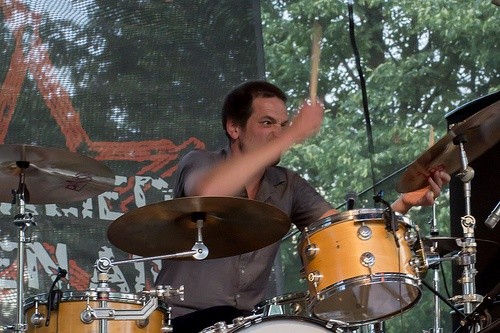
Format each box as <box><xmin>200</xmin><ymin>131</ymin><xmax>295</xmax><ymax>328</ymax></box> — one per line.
<box><xmin>297</xmin><ymin>207</ymin><xmax>423</xmax><ymax>326</ymax></box>
<box><xmin>198</xmin><ymin>312</ymin><xmax>356</xmax><ymax>333</ymax></box>
<box><xmin>23</xmin><ymin>289</ymin><xmax>172</xmax><ymax>333</ymax></box>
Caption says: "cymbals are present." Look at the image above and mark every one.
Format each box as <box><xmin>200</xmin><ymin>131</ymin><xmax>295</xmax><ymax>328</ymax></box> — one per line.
<box><xmin>421</xmin><ymin>237</ymin><xmax>500</xmax><ymax>254</ymax></box>
<box><xmin>107</xmin><ymin>195</ymin><xmax>291</xmax><ymax>261</ymax></box>
<box><xmin>396</xmin><ymin>98</ymin><xmax>500</xmax><ymax>195</ymax></box>
<box><xmin>0</xmin><ymin>143</ymin><xmax>116</xmax><ymax>204</ymax></box>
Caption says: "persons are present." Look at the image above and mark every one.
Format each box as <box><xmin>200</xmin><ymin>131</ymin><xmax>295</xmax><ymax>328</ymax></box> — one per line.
<box><xmin>153</xmin><ymin>79</ymin><xmax>450</xmax><ymax>333</ymax></box>
